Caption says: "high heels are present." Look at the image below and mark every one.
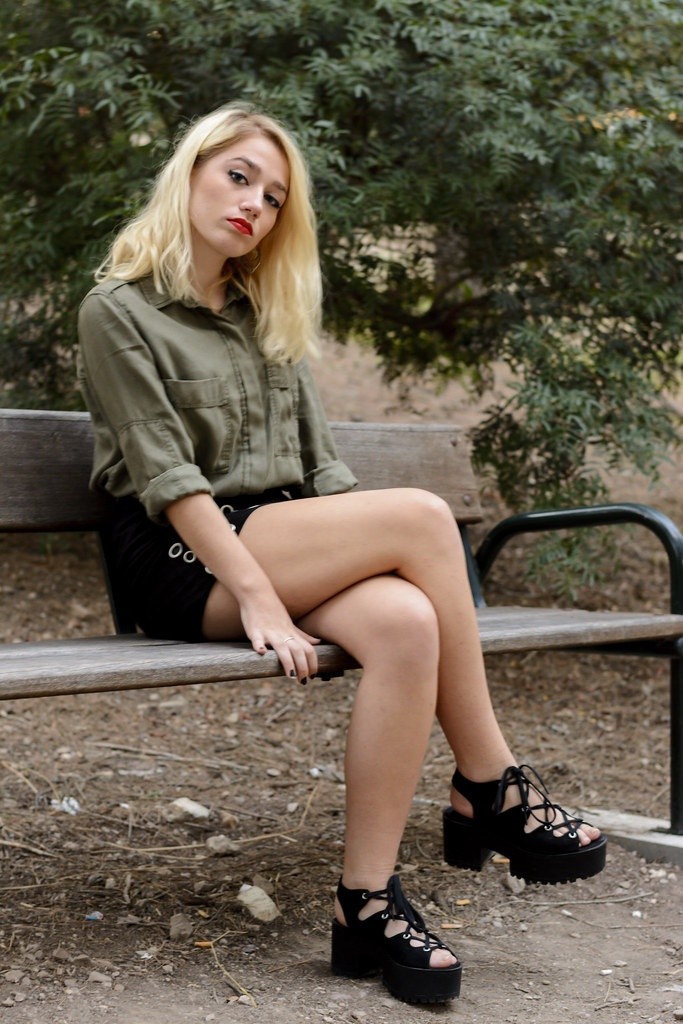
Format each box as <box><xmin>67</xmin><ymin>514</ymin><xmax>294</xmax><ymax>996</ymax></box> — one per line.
<box><xmin>442</xmin><ymin>767</ymin><xmax>608</xmax><ymax>886</ymax></box>
<box><xmin>330</xmin><ymin>874</ymin><xmax>462</xmax><ymax>1007</ymax></box>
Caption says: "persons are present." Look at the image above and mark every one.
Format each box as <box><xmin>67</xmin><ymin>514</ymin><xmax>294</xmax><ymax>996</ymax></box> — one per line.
<box><xmin>74</xmin><ymin>97</ymin><xmax>610</xmax><ymax>1008</ymax></box>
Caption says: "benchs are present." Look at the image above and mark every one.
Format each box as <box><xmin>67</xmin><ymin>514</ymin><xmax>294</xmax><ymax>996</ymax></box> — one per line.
<box><xmin>0</xmin><ymin>408</ymin><xmax>682</xmax><ymax>834</ymax></box>
